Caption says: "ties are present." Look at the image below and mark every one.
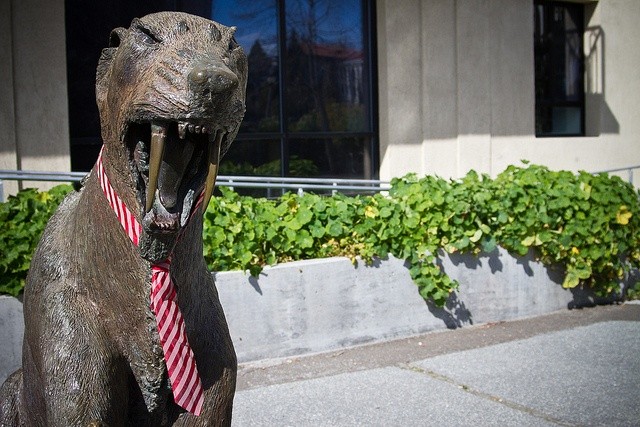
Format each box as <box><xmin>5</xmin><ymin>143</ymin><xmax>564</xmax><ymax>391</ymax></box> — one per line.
<box><xmin>96</xmin><ymin>143</ymin><xmax>206</xmax><ymax>418</ymax></box>
